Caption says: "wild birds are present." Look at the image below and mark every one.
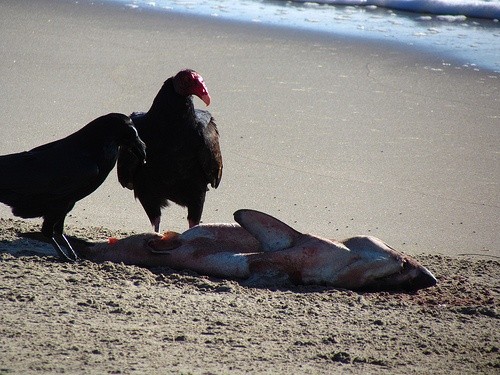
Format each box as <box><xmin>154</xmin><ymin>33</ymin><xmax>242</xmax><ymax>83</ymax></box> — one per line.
<box><xmin>0</xmin><ymin>113</ymin><xmax>147</xmax><ymax>269</ymax></box>
<box><xmin>116</xmin><ymin>69</ymin><xmax>224</xmax><ymax>232</ymax></box>
